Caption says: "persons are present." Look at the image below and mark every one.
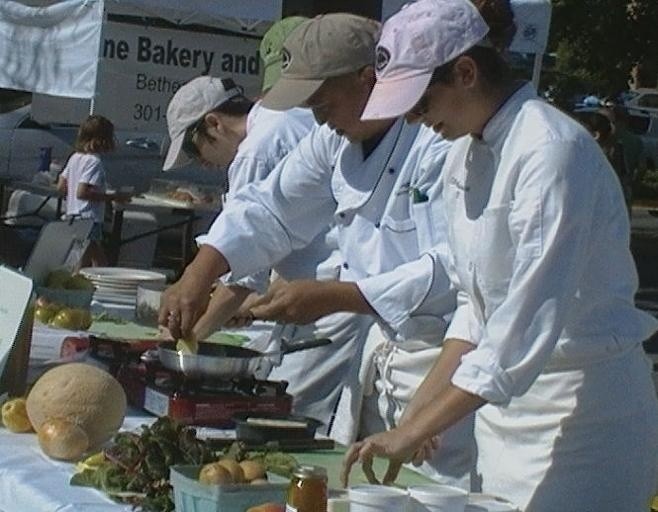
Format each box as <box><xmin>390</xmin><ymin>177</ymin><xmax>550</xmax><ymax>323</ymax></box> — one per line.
<box><xmin>160</xmin><ymin>76</ymin><xmax>359</xmax><ymax>444</ymax></box>
<box><xmin>258</xmin><ymin>14</ymin><xmax>314</xmax><ymax>90</ymax></box>
<box><xmin>157</xmin><ymin>2</ymin><xmax>480</xmax><ymax>511</ymax></box>
<box><xmin>339</xmin><ymin>3</ymin><xmax>657</xmax><ymax>511</ymax></box>
<box><xmin>56</xmin><ymin>115</ymin><xmax>133</xmax><ymax>268</ymax></box>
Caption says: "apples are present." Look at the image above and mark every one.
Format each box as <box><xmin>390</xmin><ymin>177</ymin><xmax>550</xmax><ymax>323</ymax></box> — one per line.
<box><xmin>1</xmin><ymin>397</ymin><xmax>33</xmax><ymax>434</ymax></box>
<box><xmin>45</xmin><ymin>270</ymin><xmax>93</xmax><ymax>289</ymax></box>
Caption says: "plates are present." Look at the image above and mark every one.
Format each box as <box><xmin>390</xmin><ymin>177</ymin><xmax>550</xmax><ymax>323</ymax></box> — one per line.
<box><xmin>77</xmin><ymin>268</ymin><xmax>167</xmax><ymax>305</ymax></box>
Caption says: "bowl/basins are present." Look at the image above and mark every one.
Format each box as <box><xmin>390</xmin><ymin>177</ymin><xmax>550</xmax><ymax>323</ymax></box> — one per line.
<box><xmin>230</xmin><ymin>411</ymin><xmax>325</xmax><ymax>443</ymax></box>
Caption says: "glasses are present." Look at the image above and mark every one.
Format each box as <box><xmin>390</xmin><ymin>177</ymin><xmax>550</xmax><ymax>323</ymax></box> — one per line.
<box><xmin>181</xmin><ymin>118</ymin><xmax>203</xmax><ymax>160</ymax></box>
<box><xmin>409</xmin><ymin>73</ymin><xmax>445</xmax><ymax>113</ymax></box>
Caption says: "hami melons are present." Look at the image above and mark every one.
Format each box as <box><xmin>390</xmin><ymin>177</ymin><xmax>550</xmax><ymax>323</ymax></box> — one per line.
<box><xmin>26</xmin><ymin>363</ymin><xmax>128</xmax><ymax>443</ymax></box>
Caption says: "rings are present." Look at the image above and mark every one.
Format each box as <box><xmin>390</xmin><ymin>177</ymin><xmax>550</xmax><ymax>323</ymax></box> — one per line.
<box><xmin>168</xmin><ymin>310</ymin><xmax>180</xmax><ymax>322</ymax></box>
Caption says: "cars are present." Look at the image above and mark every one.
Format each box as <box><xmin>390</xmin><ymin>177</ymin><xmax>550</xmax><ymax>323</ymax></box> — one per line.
<box><xmin>0</xmin><ymin>102</ymin><xmax>228</xmax><ymax>191</ymax></box>
<box><xmin>574</xmin><ymin>86</ymin><xmax>657</xmax><ymax>166</ymax></box>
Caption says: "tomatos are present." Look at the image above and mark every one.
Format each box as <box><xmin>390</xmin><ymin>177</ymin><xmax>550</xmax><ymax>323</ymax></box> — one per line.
<box><xmin>35</xmin><ymin>297</ymin><xmax>94</xmax><ymax>331</ymax></box>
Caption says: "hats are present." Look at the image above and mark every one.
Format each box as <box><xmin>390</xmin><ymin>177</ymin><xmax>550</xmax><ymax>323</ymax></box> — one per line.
<box><xmin>260</xmin><ymin>16</ymin><xmax>310</xmax><ymax>93</ymax></box>
<box><xmin>260</xmin><ymin>13</ymin><xmax>382</xmax><ymax>110</ymax></box>
<box><xmin>163</xmin><ymin>77</ymin><xmax>242</xmax><ymax>171</ymax></box>
<box><xmin>360</xmin><ymin>0</ymin><xmax>490</xmax><ymax>121</ymax></box>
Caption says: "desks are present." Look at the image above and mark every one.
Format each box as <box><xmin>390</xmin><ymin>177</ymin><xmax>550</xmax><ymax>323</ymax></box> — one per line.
<box><xmin>1</xmin><ymin>178</ymin><xmax>195</xmax><ymax>273</ymax></box>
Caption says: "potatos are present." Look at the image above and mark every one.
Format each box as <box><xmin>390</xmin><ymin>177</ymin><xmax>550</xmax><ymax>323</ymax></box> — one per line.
<box><xmin>246</xmin><ymin>501</ymin><xmax>284</xmax><ymax>511</ymax></box>
<box><xmin>38</xmin><ymin>420</ymin><xmax>89</xmax><ymax>460</ymax></box>
<box><xmin>199</xmin><ymin>457</ymin><xmax>272</xmax><ymax>485</ymax></box>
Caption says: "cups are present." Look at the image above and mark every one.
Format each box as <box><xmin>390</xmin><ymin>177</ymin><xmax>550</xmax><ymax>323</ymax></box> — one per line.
<box><xmin>347</xmin><ymin>484</ymin><xmax>467</xmax><ymax>512</ymax></box>
<box><xmin>136</xmin><ymin>283</ymin><xmax>165</xmax><ymax>323</ymax></box>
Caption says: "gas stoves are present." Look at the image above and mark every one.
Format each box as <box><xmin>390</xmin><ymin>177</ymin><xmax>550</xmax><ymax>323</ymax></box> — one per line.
<box><xmin>61</xmin><ymin>336</ymin><xmax>293</xmax><ymax>426</ymax></box>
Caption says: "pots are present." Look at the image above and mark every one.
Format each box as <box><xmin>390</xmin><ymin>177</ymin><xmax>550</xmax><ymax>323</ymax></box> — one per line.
<box><xmin>159</xmin><ymin>336</ymin><xmax>332</xmax><ymax>386</ymax></box>
